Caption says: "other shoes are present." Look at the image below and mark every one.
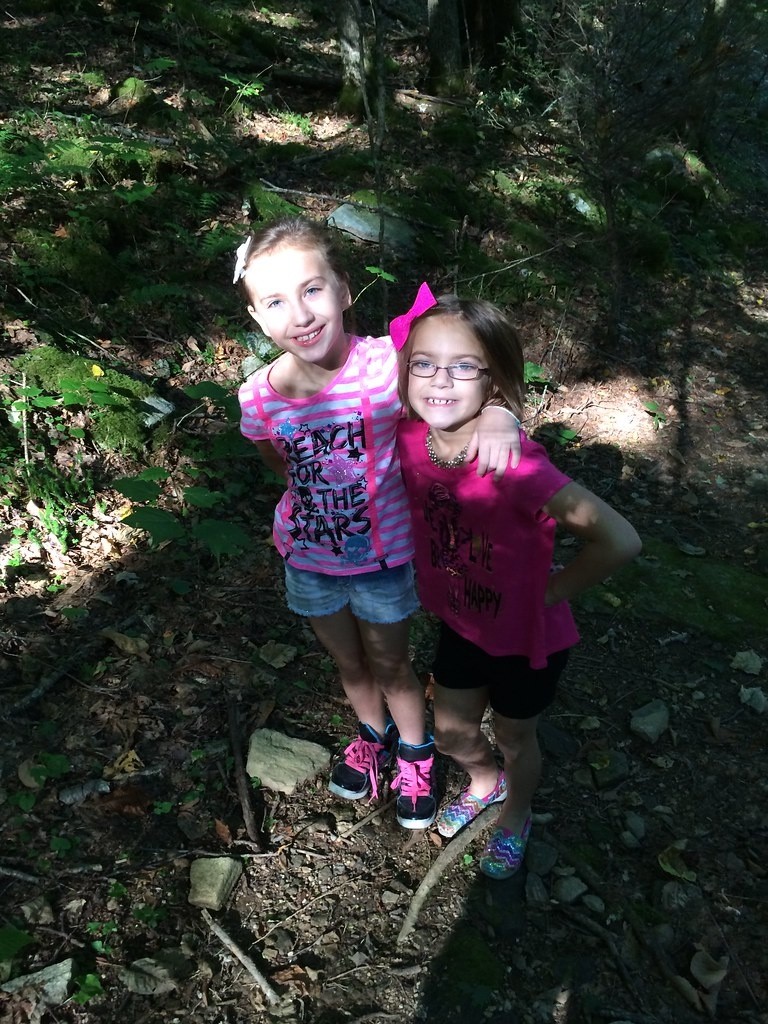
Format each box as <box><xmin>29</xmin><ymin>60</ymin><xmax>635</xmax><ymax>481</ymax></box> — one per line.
<box><xmin>435</xmin><ymin>764</ymin><xmax>510</xmax><ymax>841</ymax></box>
<box><xmin>478</xmin><ymin>795</ymin><xmax>534</xmax><ymax>882</ymax></box>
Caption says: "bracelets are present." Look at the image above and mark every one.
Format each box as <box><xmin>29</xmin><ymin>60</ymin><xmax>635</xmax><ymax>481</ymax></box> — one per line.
<box><xmin>548</xmin><ymin>563</ymin><xmax>565</xmax><ymax>579</ymax></box>
<box><xmin>481</xmin><ymin>403</ymin><xmax>522</xmax><ymax>426</ymax></box>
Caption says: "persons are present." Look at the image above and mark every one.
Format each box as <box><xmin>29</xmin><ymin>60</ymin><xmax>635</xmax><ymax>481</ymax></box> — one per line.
<box><xmin>231</xmin><ymin>218</ymin><xmax>523</xmax><ymax>830</ymax></box>
<box><xmin>392</xmin><ymin>291</ymin><xmax>643</xmax><ymax>882</ymax></box>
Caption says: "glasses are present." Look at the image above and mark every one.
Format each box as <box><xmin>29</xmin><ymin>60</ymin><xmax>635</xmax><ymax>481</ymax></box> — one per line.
<box><xmin>404</xmin><ymin>358</ymin><xmax>497</xmax><ymax>384</ymax></box>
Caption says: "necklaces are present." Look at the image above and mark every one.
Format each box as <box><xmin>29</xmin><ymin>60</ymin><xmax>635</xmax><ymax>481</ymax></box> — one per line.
<box><xmin>425</xmin><ymin>426</ymin><xmax>472</xmax><ymax>471</ymax></box>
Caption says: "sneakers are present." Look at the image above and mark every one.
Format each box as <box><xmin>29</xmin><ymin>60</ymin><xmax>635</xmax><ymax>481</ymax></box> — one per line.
<box><xmin>388</xmin><ymin>730</ymin><xmax>442</xmax><ymax>831</ymax></box>
<box><xmin>327</xmin><ymin>715</ymin><xmax>397</xmax><ymax>801</ymax></box>
